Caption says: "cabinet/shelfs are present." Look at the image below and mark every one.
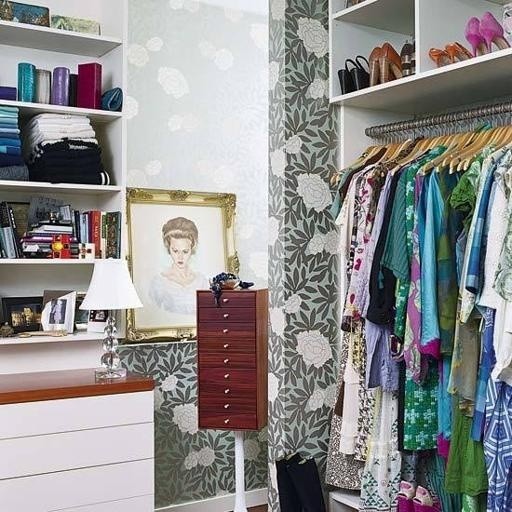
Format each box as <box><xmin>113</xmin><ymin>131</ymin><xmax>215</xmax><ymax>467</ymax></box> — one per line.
<box><xmin>0</xmin><ymin>2</ymin><xmax>129</xmax><ymax>344</ymax></box>
<box><xmin>326</xmin><ymin>2</ymin><xmax>512</xmax><ymax>105</ymax></box>
<box><xmin>196</xmin><ymin>291</ymin><xmax>257</xmax><ymax>430</ymax></box>
<box><xmin>0</xmin><ymin>391</ymin><xmax>155</xmax><ymax>512</ymax></box>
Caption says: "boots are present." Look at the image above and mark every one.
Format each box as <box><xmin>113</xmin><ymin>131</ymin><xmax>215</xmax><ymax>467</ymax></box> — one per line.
<box><xmin>275</xmin><ymin>453</ymin><xmax>326</xmax><ymax>512</ymax></box>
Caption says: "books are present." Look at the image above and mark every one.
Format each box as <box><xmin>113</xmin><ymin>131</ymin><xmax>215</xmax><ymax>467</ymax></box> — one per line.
<box><xmin>0</xmin><ymin>195</ymin><xmax>121</xmax><ymax>259</ymax></box>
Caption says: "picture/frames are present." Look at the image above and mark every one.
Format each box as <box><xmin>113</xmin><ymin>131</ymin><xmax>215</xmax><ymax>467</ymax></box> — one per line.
<box><xmin>0</xmin><ymin>289</ymin><xmax>113</xmax><ymax>337</ymax></box>
<box><xmin>122</xmin><ymin>185</ymin><xmax>238</xmax><ymax>347</ymax></box>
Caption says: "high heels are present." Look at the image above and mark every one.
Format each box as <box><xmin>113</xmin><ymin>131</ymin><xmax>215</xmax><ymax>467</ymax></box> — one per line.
<box><xmin>398</xmin><ymin>481</ymin><xmax>414</xmax><ymax>511</ymax></box>
<box><xmin>429</xmin><ymin>48</ymin><xmax>456</xmax><ymax>68</ymax></box>
<box><xmin>446</xmin><ymin>42</ymin><xmax>472</xmax><ymax>64</ymax></box>
<box><xmin>465</xmin><ymin>17</ymin><xmax>487</xmax><ymax>57</ymax></box>
<box><xmin>479</xmin><ymin>12</ymin><xmax>509</xmax><ymax>53</ymax></box>
<box><xmin>414</xmin><ymin>485</ymin><xmax>441</xmax><ymax>512</ymax></box>
<box><xmin>338</xmin><ymin>42</ymin><xmax>415</xmax><ymax>95</ymax></box>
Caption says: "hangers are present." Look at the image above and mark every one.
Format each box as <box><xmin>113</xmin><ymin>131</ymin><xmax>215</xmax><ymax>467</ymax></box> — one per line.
<box><xmin>340</xmin><ymin>107</ymin><xmax>512</xmax><ymax>175</ymax></box>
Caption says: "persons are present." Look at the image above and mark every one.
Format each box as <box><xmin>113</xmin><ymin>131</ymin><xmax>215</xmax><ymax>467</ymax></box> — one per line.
<box><xmin>51</xmin><ymin>298</ymin><xmax>62</xmax><ymax>325</ymax></box>
<box><xmin>141</xmin><ymin>218</ymin><xmax>212</xmax><ymax>317</ymax></box>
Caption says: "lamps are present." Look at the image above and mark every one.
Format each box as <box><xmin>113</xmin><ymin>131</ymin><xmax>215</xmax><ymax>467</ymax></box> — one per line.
<box><xmin>78</xmin><ymin>260</ymin><xmax>144</xmax><ymax>381</ymax></box>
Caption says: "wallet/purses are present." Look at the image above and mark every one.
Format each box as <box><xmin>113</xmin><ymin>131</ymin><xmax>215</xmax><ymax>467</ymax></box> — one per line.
<box><xmin>101</xmin><ymin>87</ymin><xmax>123</xmax><ymax>109</ymax></box>
